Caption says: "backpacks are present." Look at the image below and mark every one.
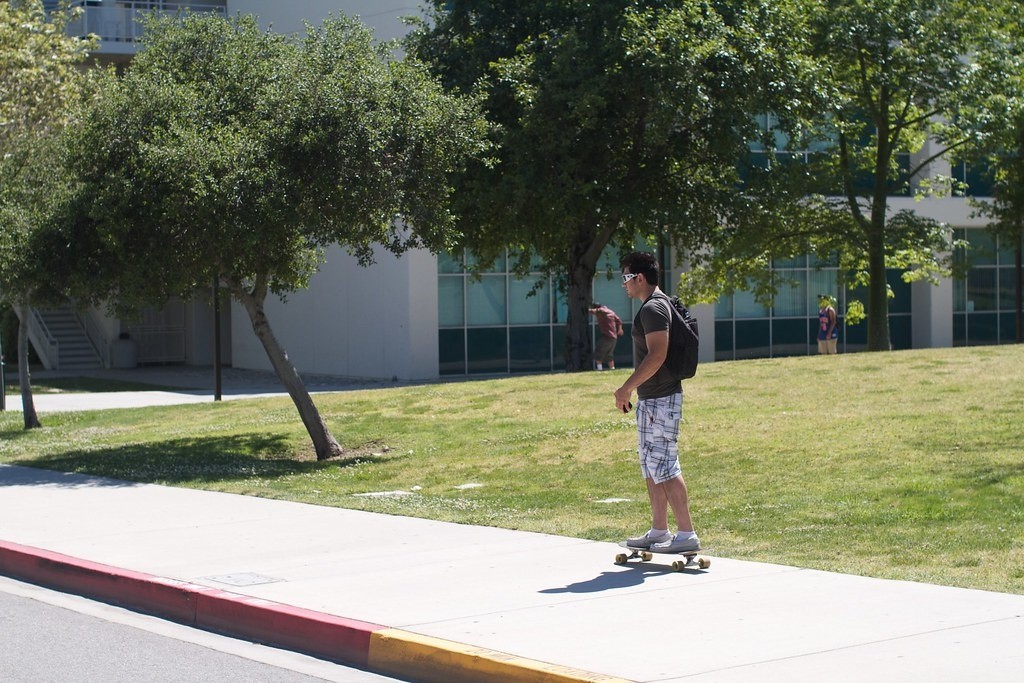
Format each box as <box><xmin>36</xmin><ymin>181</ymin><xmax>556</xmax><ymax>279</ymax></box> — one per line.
<box><xmin>633</xmin><ymin>295</ymin><xmax>699</xmax><ymax>380</ymax></box>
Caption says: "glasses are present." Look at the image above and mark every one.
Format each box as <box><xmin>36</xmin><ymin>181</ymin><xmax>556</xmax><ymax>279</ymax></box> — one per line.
<box><xmin>622</xmin><ymin>273</ymin><xmax>637</xmax><ymax>283</ymax></box>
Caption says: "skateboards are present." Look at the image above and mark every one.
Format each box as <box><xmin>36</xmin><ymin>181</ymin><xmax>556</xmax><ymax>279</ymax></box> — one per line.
<box><xmin>614</xmin><ymin>541</ymin><xmax>717</xmax><ymax>573</ymax></box>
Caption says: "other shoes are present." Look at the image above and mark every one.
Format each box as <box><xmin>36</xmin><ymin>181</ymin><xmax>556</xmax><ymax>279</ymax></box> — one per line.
<box><xmin>626</xmin><ymin>530</ymin><xmax>672</xmax><ymax>547</ymax></box>
<box><xmin>650</xmin><ymin>534</ymin><xmax>699</xmax><ymax>553</ymax></box>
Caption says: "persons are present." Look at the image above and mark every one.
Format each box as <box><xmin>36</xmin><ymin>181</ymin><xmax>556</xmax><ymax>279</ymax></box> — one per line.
<box><xmin>816</xmin><ymin>294</ymin><xmax>838</xmax><ymax>354</ymax></box>
<box><xmin>613</xmin><ymin>251</ymin><xmax>701</xmax><ymax>553</ymax></box>
<box><xmin>588</xmin><ymin>301</ymin><xmax>625</xmax><ymax>371</ymax></box>
